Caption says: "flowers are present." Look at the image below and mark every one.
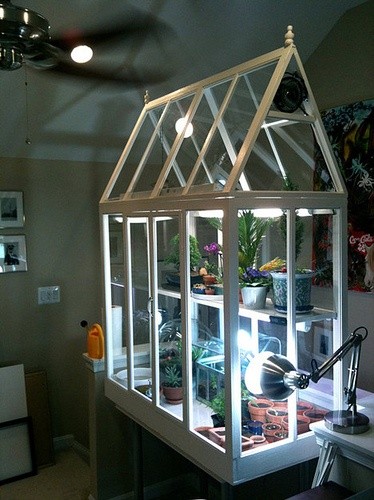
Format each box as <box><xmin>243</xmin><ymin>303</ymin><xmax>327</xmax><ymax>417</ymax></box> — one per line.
<box><xmin>242</xmin><ymin>266</ymin><xmax>269</xmax><ymax>282</ymax></box>
<box><xmin>204</xmin><ymin>243</ymin><xmax>220</xmax><ymax>253</ymax></box>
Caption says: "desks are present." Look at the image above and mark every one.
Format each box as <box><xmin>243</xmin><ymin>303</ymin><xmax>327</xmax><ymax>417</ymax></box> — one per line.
<box><xmin>309</xmin><ymin>395</ymin><xmax>374</xmax><ymax>500</ymax></box>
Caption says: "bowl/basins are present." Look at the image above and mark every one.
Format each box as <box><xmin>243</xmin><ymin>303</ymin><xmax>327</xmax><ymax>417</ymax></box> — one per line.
<box><xmin>116</xmin><ymin>367</ymin><xmax>152</xmax><ymax>388</ymax></box>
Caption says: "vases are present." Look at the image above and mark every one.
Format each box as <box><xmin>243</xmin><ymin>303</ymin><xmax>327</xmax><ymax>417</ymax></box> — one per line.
<box><xmin>242</xmin><ymin>286</ymin><xmax>267</xmax><ymax>309</ymax></box>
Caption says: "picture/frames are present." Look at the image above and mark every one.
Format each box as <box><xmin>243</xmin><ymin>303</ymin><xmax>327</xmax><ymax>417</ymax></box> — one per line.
<box><xmin>0</xmin><ymin>189</ymin><xmax>25</xmax><ymax>229</ymax></box>
<box><xmin>0</xmin><ymin>234</ymin><xmax>27</xmax><ymax>274</ymax></box>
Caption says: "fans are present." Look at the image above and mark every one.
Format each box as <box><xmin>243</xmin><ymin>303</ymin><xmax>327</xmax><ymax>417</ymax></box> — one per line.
<box><xmin>0</xmin><ymin>0</ymin><xmax>179</xmax><ymax>146</ymax></box>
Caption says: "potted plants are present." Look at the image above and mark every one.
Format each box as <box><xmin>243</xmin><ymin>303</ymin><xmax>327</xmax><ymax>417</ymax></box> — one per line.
<box><xmin>159</xmin><ymin>209</ymin><xmax>330</xmax><ymax>452</ymax></box>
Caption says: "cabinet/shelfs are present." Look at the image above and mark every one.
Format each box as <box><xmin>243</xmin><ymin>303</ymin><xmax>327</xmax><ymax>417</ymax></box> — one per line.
<box><xmin>99</xmin><ymin>25</ymin><xmax>348</xmax><ymax>500</ymax></box>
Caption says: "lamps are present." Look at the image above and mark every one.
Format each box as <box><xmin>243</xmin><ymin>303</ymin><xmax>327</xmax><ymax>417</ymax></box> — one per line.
<box><xmin>260</xmin><ymin>326</ymin><xmax>369</xmax><ymax>434</ymax></box>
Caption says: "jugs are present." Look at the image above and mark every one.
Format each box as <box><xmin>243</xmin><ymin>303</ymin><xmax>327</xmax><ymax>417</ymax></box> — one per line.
<box><xmin>80</xmin><ymin>320</ymin><xmax>104</xmax><ymax>359</ymax></box>
